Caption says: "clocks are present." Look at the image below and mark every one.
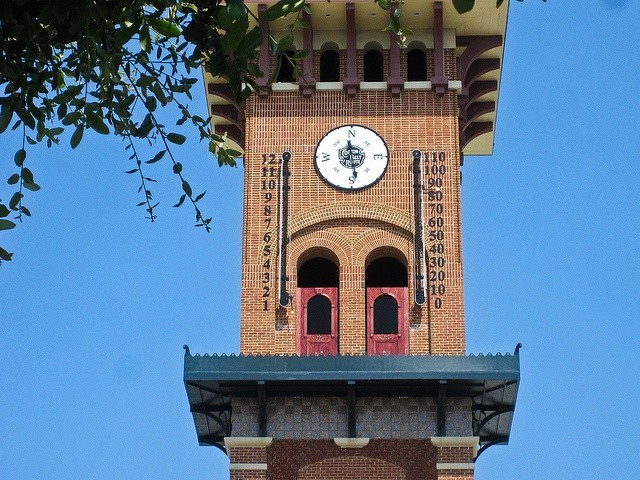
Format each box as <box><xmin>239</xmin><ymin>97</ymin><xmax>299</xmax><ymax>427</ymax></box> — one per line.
<box><xmin>314</xmin><ymin>124</ymin><xmax>390</xmax><ymax>192</ymax></box>
